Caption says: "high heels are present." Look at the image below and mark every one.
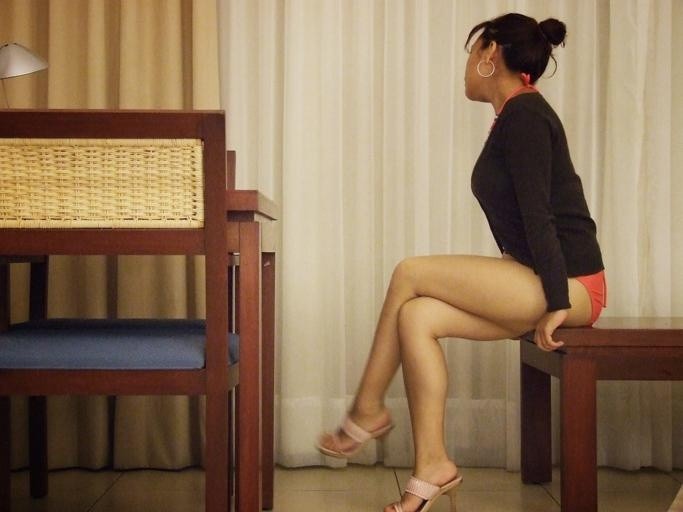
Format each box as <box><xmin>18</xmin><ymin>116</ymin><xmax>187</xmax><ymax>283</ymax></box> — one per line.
<box><xmin>316</xmin><ymin>421</ymin><xmax>395</xmax><ymax>466</ymax></box>
<box><xmin>383</xmin><ymin>475</ymin><xmax>463</xmax><ymax>511</ymax></box>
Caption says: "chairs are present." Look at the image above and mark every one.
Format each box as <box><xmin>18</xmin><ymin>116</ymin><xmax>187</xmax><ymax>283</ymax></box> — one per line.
<box><xmin>2</xmin><ymin>108</ymin><xmax>240</xmax><ymax>512</ymax></box>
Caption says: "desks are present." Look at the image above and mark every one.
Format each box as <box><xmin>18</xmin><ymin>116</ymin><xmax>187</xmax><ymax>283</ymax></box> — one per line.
<box><xmin>221</xmin><ymin>189</ymin><xmax>282</xmax><ymax>511</ymax></box>
<box><xmin>520</xmin><ymin>314</ymin><xmax>680</xmax><ymax>512</ymax></box>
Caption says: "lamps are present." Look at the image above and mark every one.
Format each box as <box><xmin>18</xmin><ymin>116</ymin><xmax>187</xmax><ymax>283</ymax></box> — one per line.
<box><xmin>0</xmin><ymin>40</ymin><xmax>47</xmax><ymax>110</ymax></box>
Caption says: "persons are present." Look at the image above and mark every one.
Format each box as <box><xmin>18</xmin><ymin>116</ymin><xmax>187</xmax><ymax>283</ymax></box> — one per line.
<box><xmin>315</xmin><ymin>15</ymin><xmax>607</xmax><ymax>512</ymax></box>
